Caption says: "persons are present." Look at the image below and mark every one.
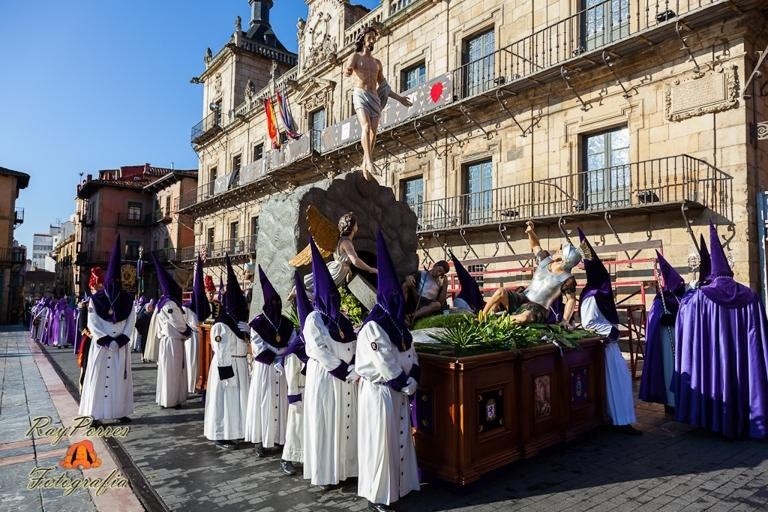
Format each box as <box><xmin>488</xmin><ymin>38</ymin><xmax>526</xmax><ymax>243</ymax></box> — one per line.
<box><xmin>343</xmin><ymin>26</ymin><xmax>413</xmax><ymax>183</ymax></box>
<box><xmin>22</xmin><ymin>211</ymin><xmax>766</xmax><ymax>508</ymax></box>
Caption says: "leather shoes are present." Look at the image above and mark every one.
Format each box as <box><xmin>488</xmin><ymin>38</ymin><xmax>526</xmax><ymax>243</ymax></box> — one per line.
<box><xmin>118</xmin><ymin>417</ymin><xmax>133</xmax><ymax>422</ymax></box>
<box><xmin>621</xmin><ymin>426</ymin><xmax>644</xmax><ymax>436</ymax></box>
<box><xmin>90</xmin><ymin>419</ymin><xmax>103</xmax><ymax>429</ymax></box>
<box><xmin>253</xmin><ymin>442</ymin><xmax>266</xmax><ymax>457</ymax></box>
<box><xmin>366</xmin><ymin>500</ymin><xmax>398</xmax><ymax>512</ymax></box>
<box><xmin>278</xmin><ymin>458</ymin><xmax>297</xmax><ymax>476</ymax></box>
<box><xmin>216</xmin><ymin>439</ymin><xmax>239</xmax><ymax>450</ymax></box>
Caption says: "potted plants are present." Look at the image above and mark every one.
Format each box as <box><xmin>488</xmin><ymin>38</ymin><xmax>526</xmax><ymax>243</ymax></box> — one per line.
<box><xmin>411</xmin><ymin>311</ymin><xmax>608</xmax><ymax>493</ymax></box>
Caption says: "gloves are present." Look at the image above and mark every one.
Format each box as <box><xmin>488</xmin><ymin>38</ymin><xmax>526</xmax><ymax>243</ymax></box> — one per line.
<box><xmin>108</xmin><ymin>341</ymin><xmax>118</xmax><ymax>352</ymax></box>
<box><xmin>236</xmin><ymin>321</ymin><xmax>250</xmax><ymax>333</ymax></box>
<box><xmin>345</xmin><ymin>365</ymin><xmax>359</xmax><ymax>383</ymax></box>
<box><xmin>290</xmin><ymin>400</ymin><xmax>303</xmax><ymax>413</ymax></box>
<box><xmin>401</xmin><ymin>377</ymin><xmax>419</xmax><ymax>396</ymax></box>
<box><xmin>226</xmin><ymin>376</ymin><xmax>237</xmax><ymax>387</ymax></box>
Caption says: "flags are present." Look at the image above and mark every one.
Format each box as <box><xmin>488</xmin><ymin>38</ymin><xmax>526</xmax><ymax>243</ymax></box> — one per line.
<box><xmin>263</xmin><ymin>83</ymin><xmax>301</xmax><ymax>150</ymax></box>
